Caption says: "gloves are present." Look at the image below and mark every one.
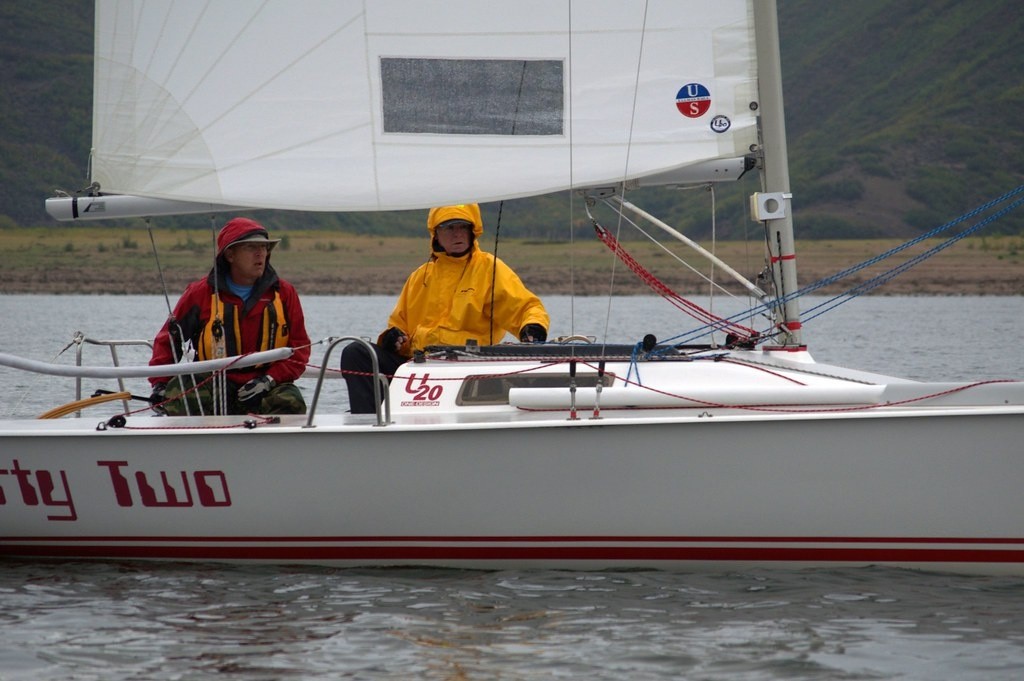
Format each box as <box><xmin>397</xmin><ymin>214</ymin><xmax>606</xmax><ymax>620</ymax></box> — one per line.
<box><xmin>238</xmin><ymin>375</ymin><xmax>274</xmax><ymax>404</ymax></box>
<box><xmin>149</xmin><ymin>384</ymin><xmax>168</xmax><ymax>415</ymax></box>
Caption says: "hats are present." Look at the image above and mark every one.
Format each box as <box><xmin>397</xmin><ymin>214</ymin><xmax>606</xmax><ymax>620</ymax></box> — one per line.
<box><xmin>438</xmin><ymin>219</ymin><xmax>472</xmax><ymax>226</ymax></box>
<box><xmin>222</xmin><ymin>234</ymin><xmax>282</xmax><ymax>255</ymax></box>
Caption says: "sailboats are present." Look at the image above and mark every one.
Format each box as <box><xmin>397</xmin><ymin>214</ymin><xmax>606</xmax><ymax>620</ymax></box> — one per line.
<box><xmin>0</xmin><ymin>0</ymin><xmax>1024</xmax><ymax>585</ymax></box>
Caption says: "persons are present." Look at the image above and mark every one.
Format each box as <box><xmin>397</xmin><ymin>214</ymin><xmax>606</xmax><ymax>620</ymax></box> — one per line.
<box><xmin>341</xmin><ymin>202</ymin><xmax>548</xmax><ymax>414</ymax></box>
<box><xmin>147</xmin><ymin>216</ymin><xmax>313</xmax><ymax>417</ymax></box>
